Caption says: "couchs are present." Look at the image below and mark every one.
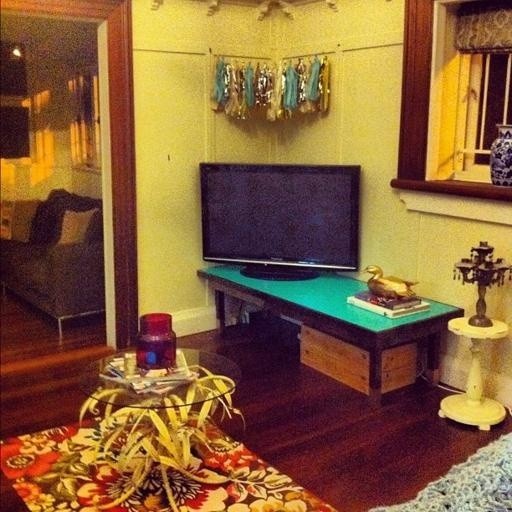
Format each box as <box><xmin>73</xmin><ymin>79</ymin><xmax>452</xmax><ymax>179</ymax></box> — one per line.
<box><xmin>0</xmin><ymin>188</ymin><xmax>105</xmax><ymax>339</ymax></box>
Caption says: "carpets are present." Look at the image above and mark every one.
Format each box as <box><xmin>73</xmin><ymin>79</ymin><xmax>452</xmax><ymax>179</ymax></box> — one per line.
<box><xmin>0</xmin><ymin>410</ymin><xmax>344</xmax><ymax>512</ymax></box>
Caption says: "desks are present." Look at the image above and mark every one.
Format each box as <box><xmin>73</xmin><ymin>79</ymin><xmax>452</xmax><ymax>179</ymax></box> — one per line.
<box><xmin>194</xmin><ymin>264</ymin><xmax>466</xmax><ymax>411</ymax></box>
<box><xmin>436</xmin><ymin>316</ymin><xmax>508</xmax><ymax>434</ymax></box>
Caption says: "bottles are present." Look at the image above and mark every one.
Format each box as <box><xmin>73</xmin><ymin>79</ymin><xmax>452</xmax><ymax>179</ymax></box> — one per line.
<box><xmin>135</xmin><ymin>313</ymin><xmax>178</xmax><ymax>370</ymax></box>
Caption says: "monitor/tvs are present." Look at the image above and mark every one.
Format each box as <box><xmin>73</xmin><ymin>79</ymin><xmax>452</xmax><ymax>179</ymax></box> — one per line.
<box><xmin>199</xmin><ymin>162</ymin><xmax>361</xmax><ymax>280</ymax></box>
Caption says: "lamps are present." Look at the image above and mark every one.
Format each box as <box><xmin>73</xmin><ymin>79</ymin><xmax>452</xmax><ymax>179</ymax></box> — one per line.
<box><xmin>452</xmin><ymin>240</ymin><xmax>512</xmax><ymax>327</ymax></box>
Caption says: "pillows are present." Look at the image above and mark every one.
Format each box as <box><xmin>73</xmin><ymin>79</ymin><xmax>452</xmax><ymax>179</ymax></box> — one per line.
<box><xmin>54</xmin><ymin>207</ymin><xmax>99</xmax><ymax>247</ymax></box>
<box><xmin>1</xmin><ymin>200</ymin><xmax>14</xmax><ymax>241</ymax></box>
<box><xmin>12</xmin><ymin>197</ymin><xmax>40</xmax><ymax>244</ymax></box>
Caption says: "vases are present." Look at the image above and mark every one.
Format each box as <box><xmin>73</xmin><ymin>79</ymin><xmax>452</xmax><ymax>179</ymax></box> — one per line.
<box><xmin>487</xmin><ymin>123</ymin><xmax>512</xmax><ymax>186</ymax></box>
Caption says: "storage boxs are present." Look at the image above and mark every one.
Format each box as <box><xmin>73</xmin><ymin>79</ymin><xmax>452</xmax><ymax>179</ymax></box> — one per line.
<box><xmin>297</xmin><ymin>322</ymin><xmax>419</xmax><ymax>397</ymax></box>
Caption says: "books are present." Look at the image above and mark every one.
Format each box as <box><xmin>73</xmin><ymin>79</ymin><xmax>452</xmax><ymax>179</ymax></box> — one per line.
<box><xmin>347</xmin><ymin>292</ymin><xmax>430</xmax><ymax>319</ymax></box>
<box><xmin>98</xmin><ymin>350</ymin><xmax>200</xmax><ymax>395</ymax></box>
